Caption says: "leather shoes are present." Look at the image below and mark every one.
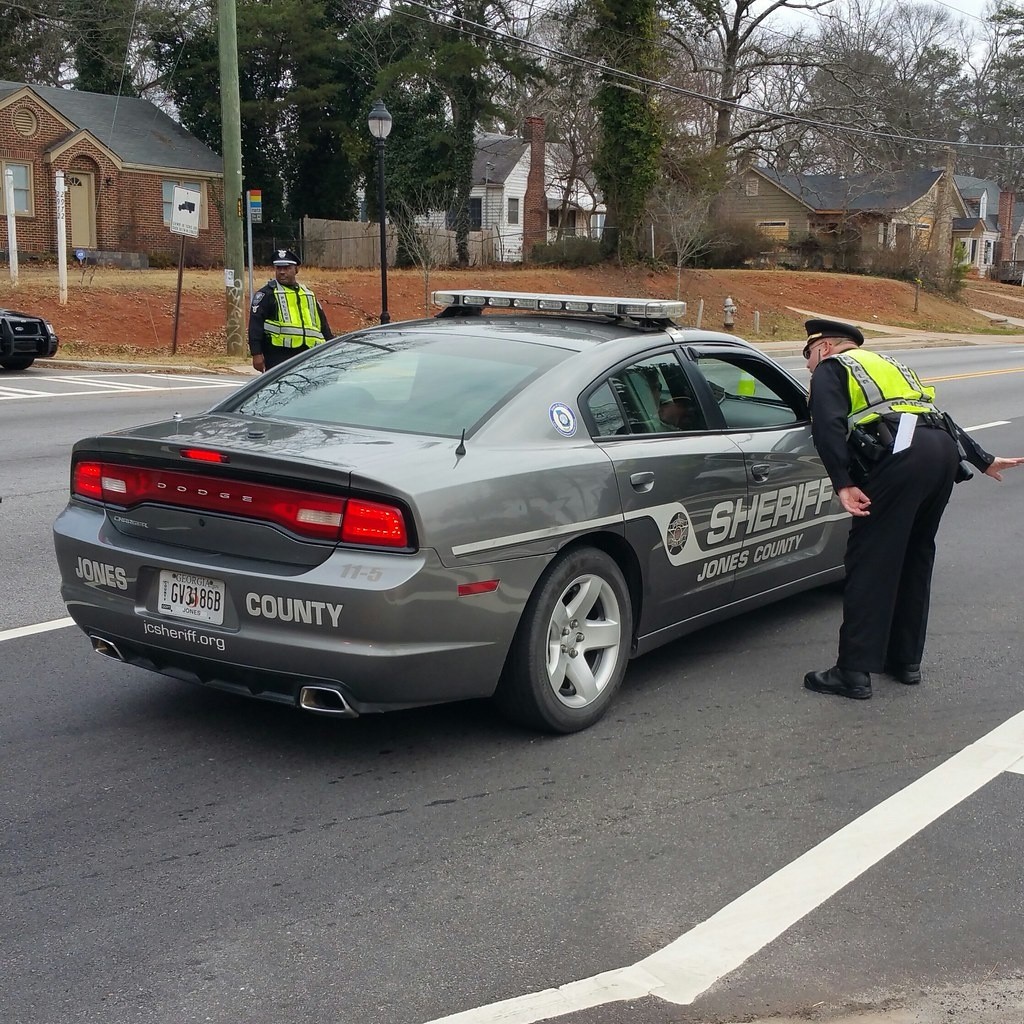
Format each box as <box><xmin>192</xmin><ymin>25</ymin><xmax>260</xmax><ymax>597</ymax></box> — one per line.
<box><xmin>883</xmin><ymin>659</ymin><xmax>921</xmax><ymax>684</ymax></box>
<box><xmin>804</xmin><ymin>663</ymin><xmax>873</xmax><ymax>699</ymax></box>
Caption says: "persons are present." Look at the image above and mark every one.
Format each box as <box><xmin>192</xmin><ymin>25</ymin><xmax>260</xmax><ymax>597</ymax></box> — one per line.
<box><xmin>248</xmin><ymin>249</ymin><xmax>334</xmax><ymax>374</ymax></box>
<box><xmin>643</xmin><ymin>367</ymin><xmax>682</xmax><ymax>431</ymax></box>
<box><xmin>803</xmin><ymin>319</ymin><xmax>1024</xmax><ymax>700</ymax></box>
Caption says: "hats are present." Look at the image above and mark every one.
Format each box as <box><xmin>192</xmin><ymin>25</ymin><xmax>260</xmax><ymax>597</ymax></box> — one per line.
<box><xmin>270</xmin><ymin>249</ymin><xmax>301</xmax><ymax>266</ymax></box>
<box><xmin>803</xmin><ymin>320</ymin><xmax>864</xmax><ymax>354</ymax></box>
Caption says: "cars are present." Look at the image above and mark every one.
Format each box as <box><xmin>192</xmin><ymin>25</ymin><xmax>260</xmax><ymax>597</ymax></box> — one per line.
<box><xmin>52</xmin><ymin>288</ymin><xmax>853</xmax><ymax>736</ymax></box>
<box><xmin>0</xmin><ymin>309</ymin><xmax>59</xmax><ymax>372</ymax></box>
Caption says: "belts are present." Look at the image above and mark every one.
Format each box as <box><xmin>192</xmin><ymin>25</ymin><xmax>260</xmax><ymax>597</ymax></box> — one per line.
<box><xmin>887</xmin><ymin>413</ymin><xmax>952</xmax><ymax>431</ymax></box>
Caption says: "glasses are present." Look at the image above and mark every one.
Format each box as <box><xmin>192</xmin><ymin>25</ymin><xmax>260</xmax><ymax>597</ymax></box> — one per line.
<box><xmin>651</xmin><ymin>384</ymin><xmax>662</xmax><ymax>393</ymax></box>
<box><xmin>803</xmin><ymin>342</ymin><xmax>825</xmax><ymax>359</ymax></box>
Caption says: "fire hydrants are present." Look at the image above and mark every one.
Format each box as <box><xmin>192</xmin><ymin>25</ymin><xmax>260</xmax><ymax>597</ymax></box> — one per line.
<box><xmin>723</xmin><ymin>295</ymin><xmax>737</xmax><ymax>331</ymax></box>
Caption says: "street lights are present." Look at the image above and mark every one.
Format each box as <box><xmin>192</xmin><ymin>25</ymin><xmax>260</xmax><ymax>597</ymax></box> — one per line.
<box><xmin>367</xmin><ymin>96</ymin><xmax>393</xmax><ymax>323</ymax></box>
